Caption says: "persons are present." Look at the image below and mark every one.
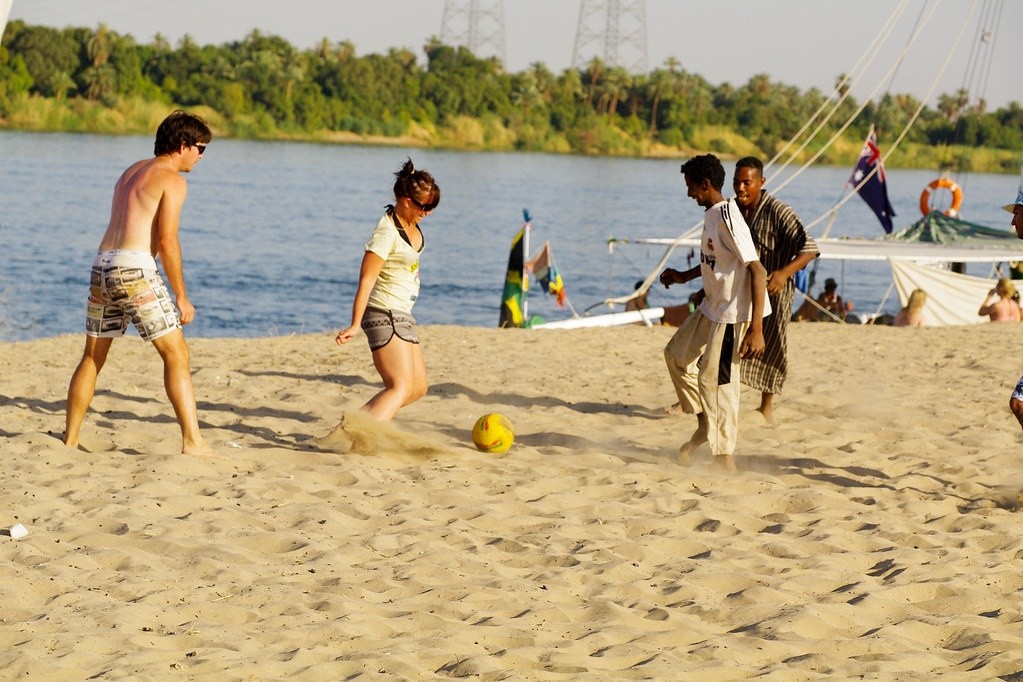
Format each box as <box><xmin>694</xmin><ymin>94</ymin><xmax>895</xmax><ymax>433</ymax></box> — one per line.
<box><xmin>336</xmin><ymin>156</ymin><xmax>441</xmax><ymax>439</ymax></box>
<box><xmin>625</xmin><ymin>280</ymin><xmax>650</xmax><ymax>310</ymax></box>
<box><xmin>794</xmin><ymin>271</ymin><xmax>853</xmax><ymax>323</ymax></box>
<box><xmin>894</xmin><ymin>289</ymin><xmax>927</xmax><ymax>328</ymax></box>
<box><xmin>659</xmin><ymin>157</ymin><xmax>821</xmax><ymax>422</ymax></box>
<box><xmin>978</xmin><ymin>279</ymin><xmax>1023</xmax><ymax>322</ymax></box>
<box><xmin>659</xmin><ymin>155</ymin><xmax>773</xmax><ymax>477</ymax></box>
<box><xmin>1001</xmin><ymin>185</ymin><xmax>1023</xmax><ymax>432</ymax></box>
<box><xmin>61</xmin><ymin>109</ymin><xmax>211</xmax><ymax>456</ymax></box>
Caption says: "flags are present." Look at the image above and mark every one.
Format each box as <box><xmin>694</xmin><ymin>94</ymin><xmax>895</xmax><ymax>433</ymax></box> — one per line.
<box><xmin>848</xmin><ymin>132</ymin><xmax>897</xmax><ymax>234</ymax></box>
<box><xmin>498</xmin><ymin>229</ymin><xmax>524</xmax><ymax>328</ymax></box>
<box><xmin>527</xmin><ymin>246</ymin><xmax>565</xmax><ymax>304</ymax></box>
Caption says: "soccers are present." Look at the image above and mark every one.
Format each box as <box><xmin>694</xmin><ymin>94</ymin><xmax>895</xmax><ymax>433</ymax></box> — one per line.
<box><xmin>472</xmin><ymin>413</ymin><xmax>515</xmax><ymax>455</ymax></box>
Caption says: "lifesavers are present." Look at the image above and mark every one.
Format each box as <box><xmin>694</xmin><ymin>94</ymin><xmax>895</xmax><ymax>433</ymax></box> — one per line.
<box><xmin>920</xmin><ymin>178</ymin><xmax>963</xmax><ymax>219</ymax></box>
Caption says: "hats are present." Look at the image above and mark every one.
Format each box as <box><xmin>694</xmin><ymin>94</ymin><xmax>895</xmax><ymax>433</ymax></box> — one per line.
<box><xmin>825</xmin><ymin>279</ymin><xmax>837</xmax><ymax>289</ymax></box>
<box><xmin>1002</xmin><ymin>189</ymin><xmax>1023</xmax><ymax>213</ymax></box>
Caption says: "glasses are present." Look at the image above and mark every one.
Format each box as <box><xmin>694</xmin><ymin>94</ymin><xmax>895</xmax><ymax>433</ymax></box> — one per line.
<box><xmin>193</xmin><ymin>143</ymin><xmax>205</xmax><ymax>154</ymax></box>
<box><xmin>410</xmin><ymin>196</ymin><xmax>434</xmax><ymax>211</ymax></box>
